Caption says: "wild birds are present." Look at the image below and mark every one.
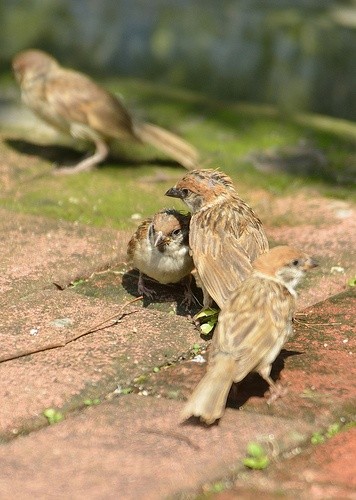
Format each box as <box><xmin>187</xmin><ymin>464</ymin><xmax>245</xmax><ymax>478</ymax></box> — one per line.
<box><xmin>126</xmin><ymin>167</ymin><xmax>321</xmax><ymax>426</ymax></box>
<box><xmin>12</xmin><ymin>48</ymin><xmax>200</xmax><ymax>178</ymax></box>
